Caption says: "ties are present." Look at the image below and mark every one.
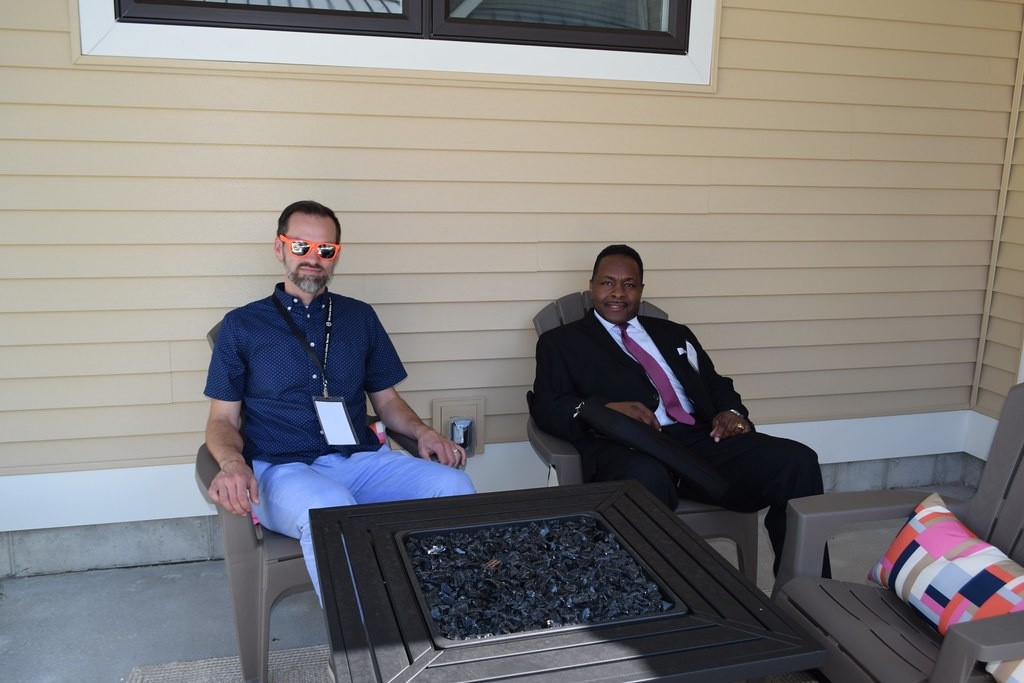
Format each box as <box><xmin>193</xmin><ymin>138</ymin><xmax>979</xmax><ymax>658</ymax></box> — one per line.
<box><xmin>616</xmin><ymin>322</ymin><xmax>696</xmax><ymax>426</ymax></box>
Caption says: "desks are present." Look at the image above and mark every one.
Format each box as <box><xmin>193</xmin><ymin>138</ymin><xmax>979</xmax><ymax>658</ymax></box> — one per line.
<box><xmin>311</xmin><ymin>479</ymin><xmax>834</xmax><ymax>683</ymax></box>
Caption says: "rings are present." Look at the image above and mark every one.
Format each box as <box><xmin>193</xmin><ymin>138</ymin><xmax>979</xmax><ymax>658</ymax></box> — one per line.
<box><xmin>736</xmin><ymin>423</ymin><xmax>744</xmax><ymax>429</ymax></box>
<box><xmin>452</xmin><ymin>449</ymin><xmax>459</xmax><ymax>453</ymax></box>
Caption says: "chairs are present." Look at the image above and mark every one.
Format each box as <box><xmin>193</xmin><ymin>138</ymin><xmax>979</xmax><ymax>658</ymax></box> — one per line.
<box><xmin>772</xmin><ymin>382</ymin><xmax>1024</xmax><ymax>683</ymax></box>
<box><xmin>526</xmin><ymin>289</ymin><xmax>760</xmax><ymax>589</ymax></box>
<box><xmin>195</xmin><ymin>321</ymin><xmax>439</xmax><ymax>683</ymax></box>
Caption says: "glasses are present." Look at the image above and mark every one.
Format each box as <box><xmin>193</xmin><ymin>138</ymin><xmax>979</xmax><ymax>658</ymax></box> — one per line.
<box><xmin>279</xmin><ymin>235</ymin><xmax>341</xmax><ymax>260</ymax></box>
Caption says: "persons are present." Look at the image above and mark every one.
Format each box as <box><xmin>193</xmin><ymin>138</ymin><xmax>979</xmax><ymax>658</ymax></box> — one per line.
<box><xmin>532</xmin><ymin>244</ymin><xmax>830</xmax><ymax>586</ymax></box>
<box><xmin>203</xmin><ymin>201</ymin><xmax>478</xmax><ymax>623</ymax></box>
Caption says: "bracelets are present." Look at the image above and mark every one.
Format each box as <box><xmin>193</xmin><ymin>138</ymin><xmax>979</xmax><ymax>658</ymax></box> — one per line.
<box><xmin>729</xmin><ymin>408</ymin><xmax>744</xmax><ymax>417</ymax></box>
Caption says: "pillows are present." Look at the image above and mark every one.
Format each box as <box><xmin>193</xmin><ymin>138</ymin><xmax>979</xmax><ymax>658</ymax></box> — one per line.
<box><xmin>866</xmin><ymin>491</ymin><xmax>1024</xmax><ymax>683</ymax></box>
